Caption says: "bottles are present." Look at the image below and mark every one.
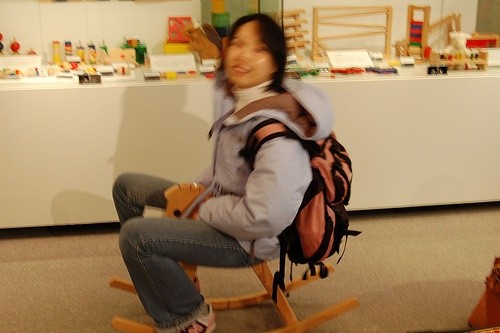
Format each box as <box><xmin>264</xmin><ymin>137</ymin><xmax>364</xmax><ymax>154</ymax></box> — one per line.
<box><xmin>51</xmin><ymin>41</ymin><xmax>98</xmax><ymax>68</ymax></box>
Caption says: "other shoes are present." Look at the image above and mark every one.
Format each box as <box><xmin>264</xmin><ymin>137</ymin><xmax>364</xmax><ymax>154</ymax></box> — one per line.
<box><xmin>176</xmin><ymin>301</ymin><xmax>218</xmax><ymax>333</ymax></box>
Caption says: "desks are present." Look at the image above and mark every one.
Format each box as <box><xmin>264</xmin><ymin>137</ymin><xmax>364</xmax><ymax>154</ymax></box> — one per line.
<box><xmin>0</xmin><ymin>49</ymin><xmax>500</xmax><ymax>230</ymax></box>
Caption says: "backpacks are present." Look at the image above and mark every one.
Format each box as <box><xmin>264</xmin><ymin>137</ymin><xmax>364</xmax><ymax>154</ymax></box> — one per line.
<box><xmin>237</xmin><ymin>116</ymin><xmax>363</xmax><ymax>306</ymax></box>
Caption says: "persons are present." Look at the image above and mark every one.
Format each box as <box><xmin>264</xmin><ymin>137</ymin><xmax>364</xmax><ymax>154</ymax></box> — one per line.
<box><xmin>112</xmin><ymin>13</ymin><xmax>333</xmax><ymax>333</ymax></box>
